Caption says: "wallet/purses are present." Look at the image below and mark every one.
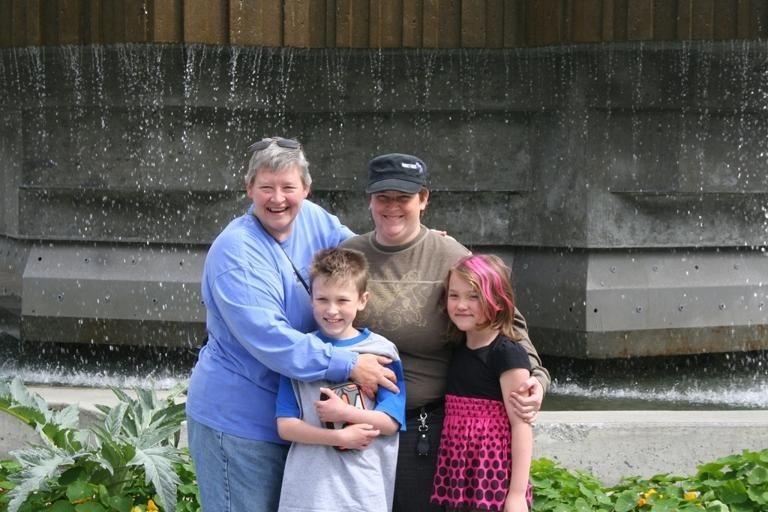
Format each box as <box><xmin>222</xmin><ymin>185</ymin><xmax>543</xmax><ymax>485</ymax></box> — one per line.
<box><xmin>320</xmin><ymin>382</ymin><xmax>366</xmax><ymax>452</ymax></box>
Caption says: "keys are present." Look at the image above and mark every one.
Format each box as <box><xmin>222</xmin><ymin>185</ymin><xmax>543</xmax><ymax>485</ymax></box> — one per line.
<box><xmin>416</xmin><ymin>433</ymin><xmax>431</xmax><ymax>456</ymax></box>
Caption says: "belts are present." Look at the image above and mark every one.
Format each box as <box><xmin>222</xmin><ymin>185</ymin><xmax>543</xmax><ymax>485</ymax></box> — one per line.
<box><xmin>405</xmin><ymin>399</ymin><xmax>445</xmax><ymax>419</ymax></box>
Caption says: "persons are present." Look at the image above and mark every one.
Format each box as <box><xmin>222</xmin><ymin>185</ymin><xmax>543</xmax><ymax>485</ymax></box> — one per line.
<box><xmin>187</xmin><ymin>136</ymin><xmax>448</xmax><ymax>511</ymax></box>
<box><xmin>275</xmin><ymin>246</ymin><xmax>409</xmax><ymax>510</ymax></box>
<box><xmin>429</xmin><ymin>253</ymin><xmax>534</xmax><ymax>512</ymax></box>
<box><xmin>337</xmin><ymin>154</ymin><xmax>551</xmax><ymax>511</ymax></box>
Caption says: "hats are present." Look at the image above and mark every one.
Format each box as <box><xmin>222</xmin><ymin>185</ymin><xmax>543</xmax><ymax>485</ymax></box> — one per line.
<box><xmin>366</xmin><ymin>153</ymin><xmax>427</xmax><ymax>193</ymax></box>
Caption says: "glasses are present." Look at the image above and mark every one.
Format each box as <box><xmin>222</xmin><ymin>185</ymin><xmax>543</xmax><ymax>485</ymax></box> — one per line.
<box><xmin>246</xmin><ymin>140</ymin><xmax>299</xmax><ymax>152</ymax></box>
